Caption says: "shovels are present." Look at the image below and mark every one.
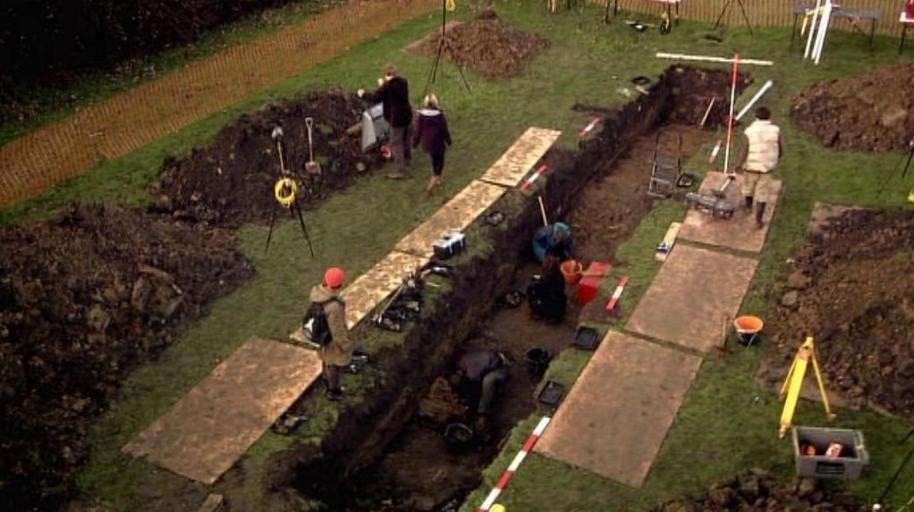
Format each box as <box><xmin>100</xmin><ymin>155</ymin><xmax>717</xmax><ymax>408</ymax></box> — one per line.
<box><xmin>709</xmin><ymin>175</ymin><xmax>735</xmax><ymax>199</ymax></box>
<box><xmin>272</xmin><ymin>126</ymin><xmax>286</xmax><ymax>175</ymax></box>
<box><xmin>305</xmin><ymin>117</ymin><xmax>321</xmax><ymax>174</ymax></box>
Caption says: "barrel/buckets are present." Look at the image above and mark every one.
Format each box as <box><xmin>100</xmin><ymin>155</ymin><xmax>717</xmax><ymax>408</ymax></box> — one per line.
<box><xmin>559</xmin><ymin>260</ymin><xmax>583</xmax><ymax>282</ymax></box>
<box><xmin>733</xmin><ymin>316</ymin><xmax>764</xmax><ymax>344</ymax></box>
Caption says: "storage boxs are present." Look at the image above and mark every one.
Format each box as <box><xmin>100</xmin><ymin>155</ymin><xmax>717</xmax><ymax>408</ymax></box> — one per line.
<box><xmin>792</xmin><ymin>422</ymin><xmax>868</xmax><ymax>481</ymax></box>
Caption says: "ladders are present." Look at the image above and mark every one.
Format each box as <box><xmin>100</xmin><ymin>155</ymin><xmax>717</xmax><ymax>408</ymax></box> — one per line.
<box><xmin>646</xmin><ymin>127</ymin><xmax>683</xmax><ymax>200</ymax></box>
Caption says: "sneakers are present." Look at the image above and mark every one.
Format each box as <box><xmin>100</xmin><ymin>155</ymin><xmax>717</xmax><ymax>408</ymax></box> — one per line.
<box><xmin>738</xmin><ymin>197</ymin><xmax>767</xmax><ymax>230</ymax></box>
<box><xmin>382</xmin><ymin>163</ymin><xmax>447</xmax><ymax>197</ymax></box>
<box><xmin>325</xmin><ymin>387</ymin><xmax>346</xmax><ymax>401</ymax></box>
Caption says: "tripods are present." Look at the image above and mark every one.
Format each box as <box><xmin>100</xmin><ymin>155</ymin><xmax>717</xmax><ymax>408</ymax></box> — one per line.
<box><xmin>265</xmin><ymin>142</ymin><xmax>315</xmax><ymax>258</ymax></box>
<box><xmin>777</xmin><ymin>338</ymin><xmax>835</xmax><ymax>438</ymax></box>
<box><xmin>422</xmin><ymin>0</ymin><xmax>472</xmax><ymax>97</ymax></box>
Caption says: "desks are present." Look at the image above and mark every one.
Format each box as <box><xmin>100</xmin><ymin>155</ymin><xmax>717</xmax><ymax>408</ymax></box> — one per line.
<box><xmin>791</xmin><ymin>5</ymin><xmax>880</xmax><ymax>51</ymax></box>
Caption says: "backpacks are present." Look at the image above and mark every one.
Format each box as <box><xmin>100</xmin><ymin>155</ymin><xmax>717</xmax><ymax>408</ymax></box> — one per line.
<box><xmin>301</xmin><ymin>295</ymin><xmax>346</xmax><ymax>346</ymax></box>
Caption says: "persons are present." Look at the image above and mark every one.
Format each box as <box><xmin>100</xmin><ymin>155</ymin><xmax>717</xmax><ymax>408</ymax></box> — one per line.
<box><xmin>449</xmin><ymin>341</ymin><xmax>507</xmax><ymax>431</ymax></box>
<box><xmin>531</xmin><ymin>222</ymin><xmax>576</xmax><ymax>264</ymax></box>
<box><xmin>356</xmin><ymin>64</ymin><xmax>414</xmax><ymax>180</ymax></box>
<box><xmin>411</xmin><ymin>92</ymin><xmax>452</xmax><ymax>196</ymax></box>
<box><xmin>524</xmin><ymin>246</ymin><xmax>568</xmax><ymax>325</ymax></box>
<box><xmin>309</xmin><ymin>266</ymin><xmax>355</xmax><ymax>402</ymax></box>
<box><xmin>733</xmin><ymin>106</ymin><xmax>785</xmax><ymax>230</ymax></box>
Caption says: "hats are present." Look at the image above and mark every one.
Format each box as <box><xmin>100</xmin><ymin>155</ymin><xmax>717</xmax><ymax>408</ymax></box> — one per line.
<box><xmin>323</xmin><ymin>266</ymin><xmax>346</xmax><ymax>287</ymax></box>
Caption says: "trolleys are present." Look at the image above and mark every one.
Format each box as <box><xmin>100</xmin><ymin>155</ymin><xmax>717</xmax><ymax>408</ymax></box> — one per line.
<box><xmin>356</xmin><ymin>90</ymin><xmax>395</xmax><ymax>163</ymax></box>
<box><xmin>784</xmin><ymin>418</ymin><xmax>871</xmax><ymax>498</ymax></box>
